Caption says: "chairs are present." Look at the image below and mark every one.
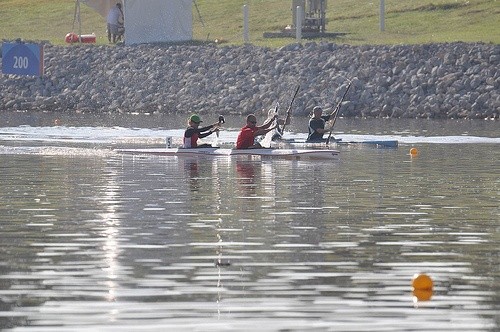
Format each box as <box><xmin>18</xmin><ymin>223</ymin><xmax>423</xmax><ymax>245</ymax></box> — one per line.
<box><xmin>116</xmin><ymin>27</ymin><xmax>124</xmax><ymax>41</ymax></box>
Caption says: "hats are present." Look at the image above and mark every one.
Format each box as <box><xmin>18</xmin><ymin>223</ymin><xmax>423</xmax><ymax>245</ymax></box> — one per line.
<box><xmin>190</xmin><ymin>115</ymin><xmax>203</xmax><ymax>122</ymax></box>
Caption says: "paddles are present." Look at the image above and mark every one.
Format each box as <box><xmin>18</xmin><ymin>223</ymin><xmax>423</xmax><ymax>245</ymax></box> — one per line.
<box><xmin>281</xmin><ymin>86</ymin><xmax>300</xmax><ymax>135</ymax></box>
<box><xmin>325</xmin><ymin>83</ymin><xmax>351</xmax><ymax>145</ymax></box>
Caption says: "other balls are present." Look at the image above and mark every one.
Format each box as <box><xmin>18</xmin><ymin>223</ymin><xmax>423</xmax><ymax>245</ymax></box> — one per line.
<box><xmin>410</xmin><ymin>148</ymin><xmax>418</xmax><ymax>155</ymax></box>
<box><xmin>413</xmin><ymin>273</ymin><xmax>432</xmax><ymax>291</ymax></box>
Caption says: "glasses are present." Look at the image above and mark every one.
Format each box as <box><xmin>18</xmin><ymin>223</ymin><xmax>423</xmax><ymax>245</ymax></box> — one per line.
<box><xmin>249</xmin><ymin>121</ymin><xmax>257</xmax><ymax>123</ymax></box>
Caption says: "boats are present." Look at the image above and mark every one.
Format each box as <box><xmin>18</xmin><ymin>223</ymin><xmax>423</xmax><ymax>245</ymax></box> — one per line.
<box><xmin>338</xmin><ymin>141</ymin><xmax>399</xmax><ymax>147</ymax></box>
<box><xmin>117</xmin><ymin>149</ymin><xmax>340</xmax><ymax>160</ymax></box>
<box><xmin>217</xmin><ymin>142</ymin><xmax>338</xmax><ymax>147</ymax></box>
